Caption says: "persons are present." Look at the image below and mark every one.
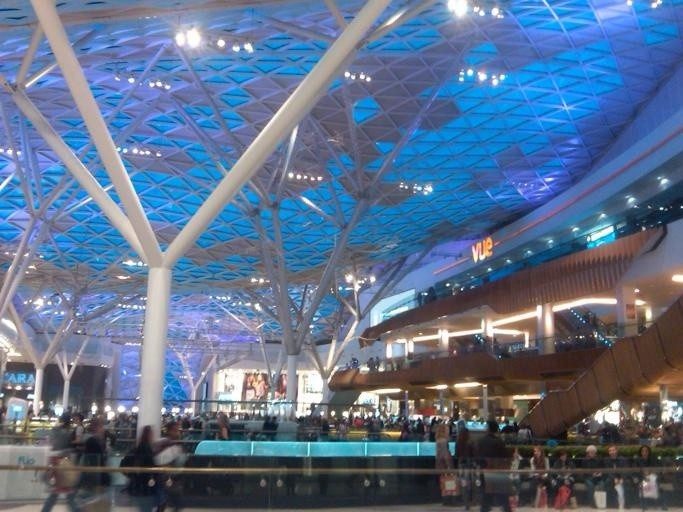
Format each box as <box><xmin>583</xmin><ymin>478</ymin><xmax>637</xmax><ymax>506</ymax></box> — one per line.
<box><xmin>0</xmin><ymin>327</ymin><xmax>683</xmax><ymax>511</ymax></box>
<box><xmin>429</xmin><ymin>287</ymin><xmax>436</xmax><ymax>303</ymax></box>
<box><xmin>584</xmin><ymin>307</ymin><xmax>598</xmax><ymax>326</ymax></box>
<box><xmin>418</xmin><ymin>290</ymin><xmax>422</xmax><ymax>308</ymax></box>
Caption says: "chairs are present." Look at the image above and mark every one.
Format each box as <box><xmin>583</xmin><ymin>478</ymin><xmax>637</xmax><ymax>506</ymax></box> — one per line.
<box><xmin>519</xmin><ymin>456</ymin><xmax>675</xmax><ymax>492</ymax></box>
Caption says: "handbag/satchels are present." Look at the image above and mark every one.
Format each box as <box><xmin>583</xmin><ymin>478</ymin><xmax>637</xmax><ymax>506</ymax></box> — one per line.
<box><xmin>554</xmin><ymin>486</ymin><xmax>571</xmax><ymax>508</ymax></box>
<box><xmin>534</xmin><ymin>486</ymin><xmax>547</xmax><ymax>508</ymax></box>
<box><xmin>439</xmin><ymin>474</ymin><xmax>460</xmax><ymax>497</ymax></box>
<box><xmin>593</xmin><ymin>491</ymin><xmax>606</xmax><ymax>509</ymax></box>
<box><xmin>636</xmin><ymin>474</ymin><xmax>658</xmax><ymax>500</ymax></box>
